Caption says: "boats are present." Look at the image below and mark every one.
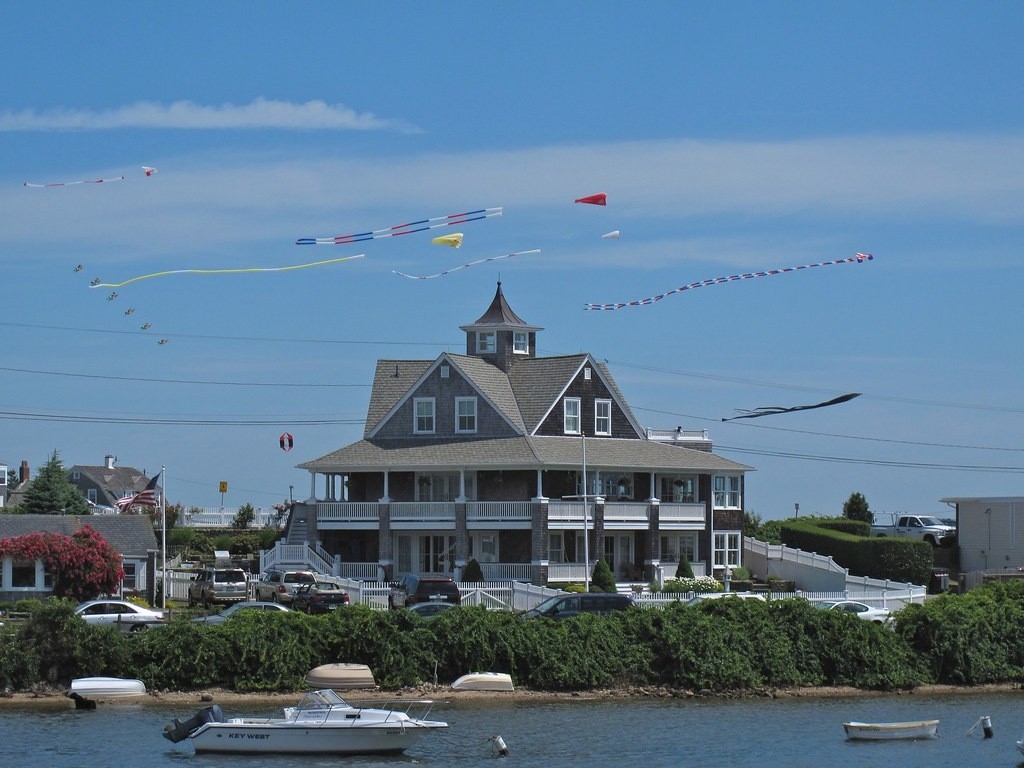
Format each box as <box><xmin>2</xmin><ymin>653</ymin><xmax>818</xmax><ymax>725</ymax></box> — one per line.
<box><xmin>842</xmin><ymin>720</ymin><xmax>939</xmax><ymax>740</ymax></box>
<box><xmin>162</xmin><ymin>689</ymin><xmax>450</xmax><ymax>756</ymax></box>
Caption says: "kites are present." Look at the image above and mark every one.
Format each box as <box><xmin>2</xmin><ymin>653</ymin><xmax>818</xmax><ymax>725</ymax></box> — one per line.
<box><xmin>74</xmin><ymin>264</ymin><xmax>168</xmax><ymax>347</ymax></box>
<box><xmin>294</xmin><ymin>192</ymin><xmax>608</xmax><ymax>245</ymax></box>
<box><xmin>582</xmin><ymin>252</ymin><xmax>873</xmax><ymax>311</ymax></box>
<box><xmin>89</xmin><ymin>233</ymin><xmax>463</xmax><ymax>290</ymax></box>
<box><xmin>23</xmin><ymin>166</ymin><xmax>160</xmax><ymax>189</ymax></box>
<box><xmin>390</xmin><ymin>228</ymin><xmax>620</xmax><ymax>280</ymax></box>
<box><xmin>720</xmin><ymin>392</ymin><xmax>863</xmax><ymax>422</ymax></box>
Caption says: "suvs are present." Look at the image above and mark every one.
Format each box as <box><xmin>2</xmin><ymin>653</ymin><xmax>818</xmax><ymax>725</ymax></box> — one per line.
<box><xmin>255</xmin><ymin>570</ymin><xmax>317</xmax><ymax>605</ymax></box>
<box><xmin>682</xmin><ymin>592</ymin><xmax>767</xmax><ymax>606</ymax></box>
<box><xmin>188</xmin><ymin>568</ymin><xmax>247</xmax><ymax>609</ymax></box>
<box><xmin>387</xmin><ymin>572</ymin><xmax>461</xmax><ymax>610</ymax></box>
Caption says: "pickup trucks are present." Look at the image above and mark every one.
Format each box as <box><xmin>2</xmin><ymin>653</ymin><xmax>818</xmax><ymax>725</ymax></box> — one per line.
<box><xmin>870</xmin><ymin>515</ymin><xmax>956</xmax><ymax>547</ymax></box>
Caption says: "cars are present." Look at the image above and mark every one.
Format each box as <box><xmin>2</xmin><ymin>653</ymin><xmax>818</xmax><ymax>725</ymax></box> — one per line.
<box><xmin>191</xmin><ymin>602</ymin><xmax>291</xmax><ymax>626</ymax></box>
<box><xmin>816</xmin><ymin>600</ymin><xmax>889</xmax><ymax>627</ymax></box>
<box><xmin>406</xmin><ymin>602</ymin><xmax>455</xmax><ymax>620</ymax></box>
<box><xmin>290</xmin><ymin>582</ymin><xmax>349</xmax><ymax>615</ymax></box>
<box><xmin>74</xmin><ymin>600</ymin><xmax>166</xmax><ymax>639</ymax></box>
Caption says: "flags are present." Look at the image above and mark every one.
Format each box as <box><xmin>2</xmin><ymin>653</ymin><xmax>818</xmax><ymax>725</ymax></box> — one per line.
<box><xmin>113</xmin><ymin>470</ymin><xmax>161</xmax><ymax>512</ymax></box>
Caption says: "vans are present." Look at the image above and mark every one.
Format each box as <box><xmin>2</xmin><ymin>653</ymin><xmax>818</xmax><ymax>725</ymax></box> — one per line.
<box><xmin>512</xmin><ymin>593</ymin><xmax>637</xmax><ymax>625</ymax></box>
